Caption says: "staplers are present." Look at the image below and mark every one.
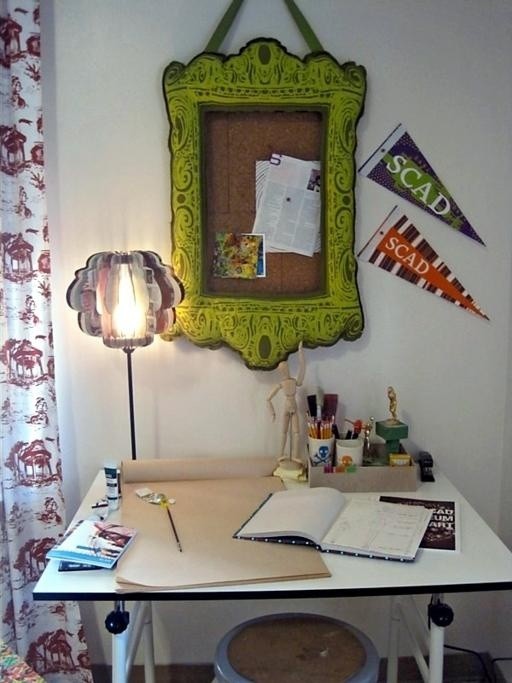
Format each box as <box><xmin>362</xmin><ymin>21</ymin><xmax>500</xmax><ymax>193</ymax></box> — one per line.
<box><xmin>418</xmin><ymin>451</ymin><xmax>436</xmax><ymax>482</ymax></box>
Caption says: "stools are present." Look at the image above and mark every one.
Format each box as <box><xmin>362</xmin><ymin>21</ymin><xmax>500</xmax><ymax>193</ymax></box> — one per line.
<box><xmin>209</xmin><ymin>611</ymin><xmax>381</xmax><ymax>683</ymax></box>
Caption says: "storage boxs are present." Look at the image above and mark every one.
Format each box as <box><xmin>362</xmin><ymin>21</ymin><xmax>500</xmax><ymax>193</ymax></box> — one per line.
<box><xmin>304</xmin><ymin>440</ymin><xmax>418</xmax><ymax>493</ymax></box>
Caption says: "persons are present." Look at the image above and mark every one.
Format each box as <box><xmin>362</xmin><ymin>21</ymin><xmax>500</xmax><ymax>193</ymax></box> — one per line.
<box><xmin>266</xmin><ymin>341</ymin><xmax>310</xmax><ymax>465</ymax></box>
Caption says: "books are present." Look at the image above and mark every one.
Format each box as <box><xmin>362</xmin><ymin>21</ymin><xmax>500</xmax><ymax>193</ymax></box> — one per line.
<box><xmin>234</xmin><ymin>487</ymin><xmax>435</xmax><ymax>562</ymax></box>
<box><xmin>45</xmin><ymin>518</ymin><xmax>137</xmax><ymax>573</ymax></box>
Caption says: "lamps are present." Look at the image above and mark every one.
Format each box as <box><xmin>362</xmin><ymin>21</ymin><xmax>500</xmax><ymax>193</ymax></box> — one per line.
<box><xmin>65</xmin><ymin>250</ymin><xmax>187</xmax><ymax>461</ymax></box>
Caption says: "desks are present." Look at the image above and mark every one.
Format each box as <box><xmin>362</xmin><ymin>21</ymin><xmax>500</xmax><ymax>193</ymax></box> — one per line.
<box><xmin>32</xmin><ymin>467</ymin><xmax>511</xmax><ymax>683</ymax></box>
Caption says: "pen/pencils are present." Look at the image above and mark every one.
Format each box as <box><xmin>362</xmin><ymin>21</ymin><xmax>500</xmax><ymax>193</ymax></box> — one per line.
<box><xmin>162</xmin><ymin>503</ymin><xmax>182</xmax><ymax>552</ymax></box>
<box><xmin>305</xmin><ymin>405</ymin><xmax>337</xmax><ymax>439</ymax></box>
<box><xmin>332</xmin><ymin>419</ymin><xmax>362</xmax><ymax>440</ymax></box>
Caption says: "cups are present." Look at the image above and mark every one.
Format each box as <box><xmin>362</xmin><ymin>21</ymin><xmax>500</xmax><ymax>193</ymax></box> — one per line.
<box><xmin>337</xmin><ymin>431</ymin><xmax>364</xmax><ymax>466</ymax></box>
<box><xmin>308</xmin><ymin>433</ymin><xmax>335</xmax><ymax>466</ymax></box>
